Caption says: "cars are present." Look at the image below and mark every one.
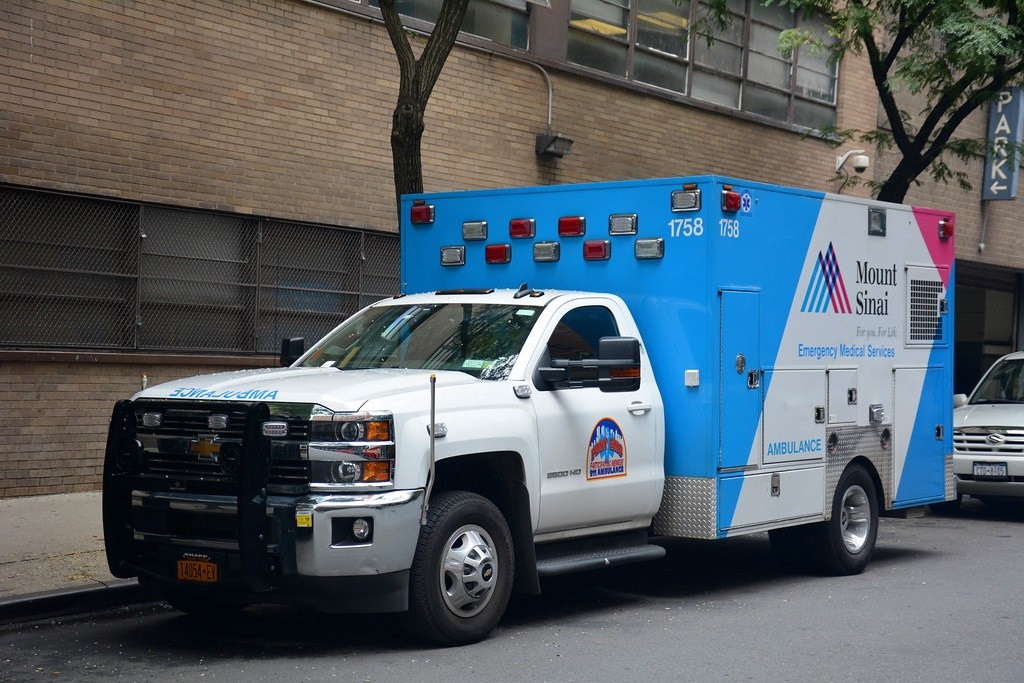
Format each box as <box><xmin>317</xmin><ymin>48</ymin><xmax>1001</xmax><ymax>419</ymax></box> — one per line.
<box><xmin>929</xmin><ymin>350</ymin><xmax>1024</xmax><ymax>513</ymax></box>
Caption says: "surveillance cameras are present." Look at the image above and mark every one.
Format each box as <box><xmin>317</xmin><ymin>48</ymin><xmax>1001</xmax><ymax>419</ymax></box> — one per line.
<box><xmin>853</xmin><ymin>155</ymin><xmax>869</xmax><ymax>173</ymax></box>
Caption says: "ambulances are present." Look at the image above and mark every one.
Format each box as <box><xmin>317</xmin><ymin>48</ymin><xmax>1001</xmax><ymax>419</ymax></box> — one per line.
<box><xmin>102</xmin><ymin>173</ymin><xmax>958</xmax><ymax>647</ymax></box>
